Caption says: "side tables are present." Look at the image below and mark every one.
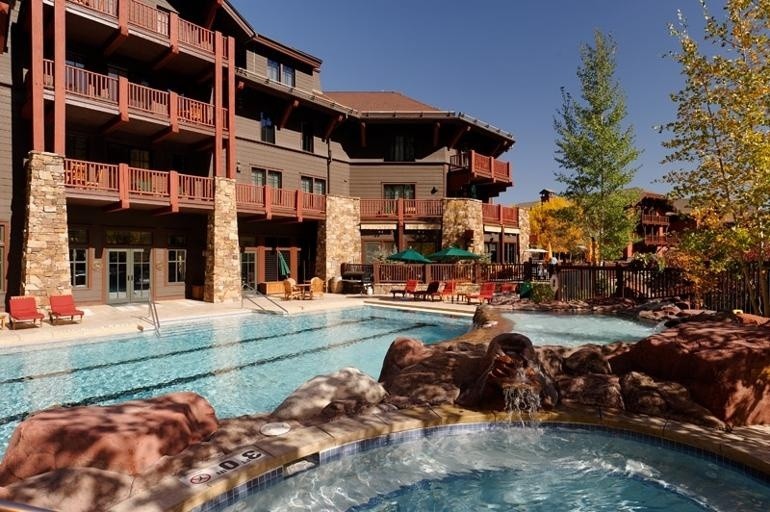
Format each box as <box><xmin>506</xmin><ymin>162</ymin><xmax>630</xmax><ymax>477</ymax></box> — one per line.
<box><xmin>0</xmin><ymin>312</ymin><xmax>9</xmax><ymax>328</ymax></box>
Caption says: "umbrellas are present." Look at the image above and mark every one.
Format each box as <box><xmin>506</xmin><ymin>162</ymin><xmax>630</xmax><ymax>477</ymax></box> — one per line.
<box><xmin>278</xmin><ymin>249</ymin><xmax>292</xmax><ymax>279</ymax></box>
<box><xmin>426</xmin><ymin>244</ymin><xmax>482</xmax><ymax>261</ymax></box>
<box><xmin>386</xmin><ymin>246</ymin><xmax>432</xmax><ymax>263</ymax></box>
<box><xmin>524</xmin><ymin>248</ymin><xmax>549</xmax><ymax>253</ymax></box>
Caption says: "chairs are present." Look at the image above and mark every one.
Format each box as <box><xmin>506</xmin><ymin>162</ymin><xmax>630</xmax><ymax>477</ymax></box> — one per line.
<box><xmin>48</xmin><ymin>295</ymin><xmax>84</xmax><ymax>324</ymax></box>
<box><xmin>284</xmin><ymin>277</ymin><xmax>323</xmax><ymax>300</ymax></box>
<box><xmin>10</xmin><ymin>296</ymin><xmax>44</xmax><ymax>330</ymax></box>
<box><xmin>390</xmin><ymin>280</ymin><xmax>516</xmax><ymax>306</ymax></box>
